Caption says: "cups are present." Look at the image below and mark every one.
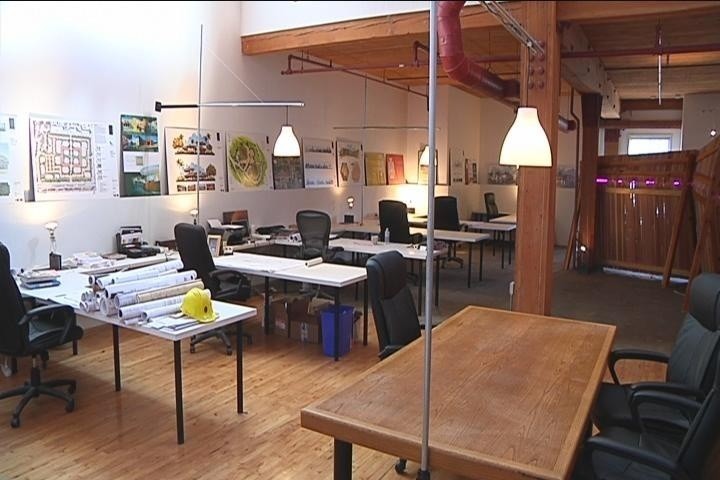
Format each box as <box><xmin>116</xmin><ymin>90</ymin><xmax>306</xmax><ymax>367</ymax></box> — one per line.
<box><xmin>371</xmin><ymin>236</ymin><xmax>378</xmax><ymax>245</ymax></box>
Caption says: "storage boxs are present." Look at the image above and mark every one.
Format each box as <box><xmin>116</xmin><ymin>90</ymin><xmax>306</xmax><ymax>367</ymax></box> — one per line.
<box><xmin>269</xmin><ymin>298</ymin><xmax>331</xmax><ymax>343</ymax></box>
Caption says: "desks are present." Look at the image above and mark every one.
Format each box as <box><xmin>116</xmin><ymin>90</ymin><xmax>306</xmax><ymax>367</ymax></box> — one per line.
<box><xmin>275</xmin><ymin>233</ymin><xmax>447</xmax><ymax>316</ymax></box>
<box><xmin>459</xmin><ymin>221</ymin><xmax>517</xmax><ymax>269</ymax></box>
<box><xmin>211</xmin><ymin>252</ymin><xmax>367</xmax><ymax>362</ymax></box>
<box><xmin>339</xmin><ymin>222</ymin><xmax>491</xmax><ymax>287</ymax></box>
<box><xmin>472</xmin><ymin>210</ymin><xmax>517</xmax><ymax>256</ymax></box>
<box><xmin>301</xmin><ymin>305</ymin><xmax>617</xmax><ymax>480</ymax></box>
<box><xmin>21</xmin><ymin>273</ymin><xmax>258</xmax><ymax>444</ymax></box>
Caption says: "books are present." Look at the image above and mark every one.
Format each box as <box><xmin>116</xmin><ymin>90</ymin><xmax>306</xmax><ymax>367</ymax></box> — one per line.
<box><xmin>16</xmin><ymin>270</ymin><xmax>60</xmax><ymax>289</ymax></box>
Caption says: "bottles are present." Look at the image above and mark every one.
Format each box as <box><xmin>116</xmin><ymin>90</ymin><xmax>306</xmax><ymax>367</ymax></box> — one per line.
<box><xmin>385</xmin><ymin>227</ymin><xmax>390</xmax><ymax>245</ymax></box>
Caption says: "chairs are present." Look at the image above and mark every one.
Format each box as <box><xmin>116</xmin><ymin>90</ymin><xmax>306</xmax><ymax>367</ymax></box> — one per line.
<box><xmin>484</xmin><ymin>192</ymin><xmax>505</xmax><ymax>244</ymax></box>
<box><xmin>174</xmin><ymin>223</ymin><xmax>253</xmax><ymax>356</ymax></box>
<box><xmin>0</xmin><ymin>242</ymin><xmax>84</xmax><ymax>428</ymax></box>
<box><xmin>573</xmin><ymin>380</ymin><xmax>720</xmax><ymax>480</ymax></box>
<box><xmin>434</xmin><ymin>196</ymin><xmax>468</xmax><ymax>268</ymax></box>
<box><xmin>592</xmin><ymin>272</ymin><xmax>720</xmax><ymax>431</ymax></box>
<box><xmin>296</xmin><ymin>210</ymin><xmax>344</xmax><ymax>300</ymax></box>
<box><xmin>377</xmin><ymin>200</ymin><xmax>423</xmax><ymax>286</ymax></box>
<box><xmin>366</xmin><ymin>251</ymin><xmax>436</xmax><ymax>360</ymax></box>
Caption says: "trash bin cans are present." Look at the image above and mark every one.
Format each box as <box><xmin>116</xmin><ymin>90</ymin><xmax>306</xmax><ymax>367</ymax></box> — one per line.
<box><xmin>321</xmin><ymin>306</ymin><xmax>353</xmax><ymax>357</ymax></box>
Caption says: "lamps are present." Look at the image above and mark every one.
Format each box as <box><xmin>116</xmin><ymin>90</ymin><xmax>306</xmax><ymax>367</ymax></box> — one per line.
<box><xmin>155</xmin><ymin>102</ymin><xmax>305</xmax><ymax>156</ymax></box>
<box><xmin>334</xmin><ymin>126</ymin><xmax>440</xmax><ymax>166</ymax></box>
<box><xmin>499</xmin><ymin>41</ymin><xmax>553</xmax><ymax>168</ymax></box>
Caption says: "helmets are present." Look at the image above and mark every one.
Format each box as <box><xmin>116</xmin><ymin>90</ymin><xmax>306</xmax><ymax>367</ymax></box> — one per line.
<box><xmin>181</xmin><ymin>287</ymin><xmax>217</xmax><ymax>323</ymax></box>
